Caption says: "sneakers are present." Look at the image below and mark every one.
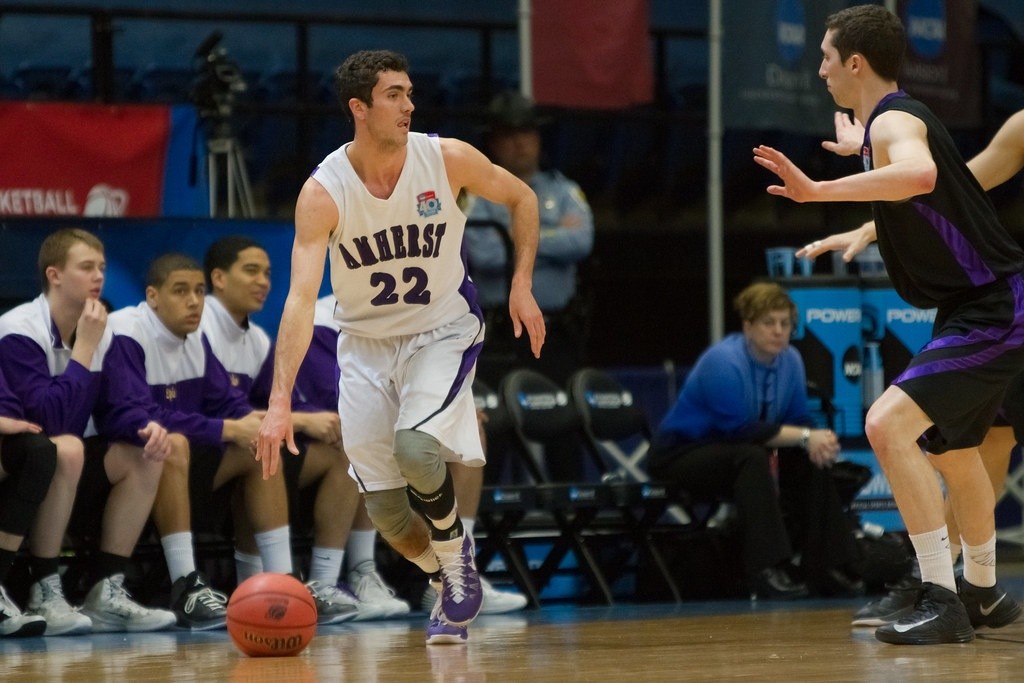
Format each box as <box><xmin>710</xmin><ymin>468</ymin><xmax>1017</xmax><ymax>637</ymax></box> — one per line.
<box><xmin>874</xmin><ymin>582</ymin><xmax>975</xmax><ymax>644</ymax></box>
<box><xmin>425</xmin><ymin>578</ymin><xmax>469</xmax><ymax>644</ymax></box>
<box><xmin>24</xmin><ymin>573</ymin><xmax>92</xmax><ymax>636</ymax></box>
<box><xmin>422</xmin><ymin>576</ymin><xmax>527</xmax><ymax>615</ymax></box>
<box><xmin>955</xmin><ymin>574</ymin><xmax>1021</xmax><ymax>629</ymax></box>
<box><xmin>0</xmin><ymin>585</ymin><xmax>47</xmax><ymax>637</ymax></box>
<box><xmin>313</xmin><ymin>585</ymin><xmax>386</xmax><ymax>621</ymax></box>
<box><xmin>170</xmin><ymin>571</ymin><xmax>227</xmax><ymax>631</ymax></box>
<box><xmin>286</xmin><ymin>567</ymin><xmax>359</xmax><ymax>624</ymax></box>
<box><xmin>850</xmin><ymin>570</ymin><xmax>922</xmax><ymax>626</ymax></box>
<box><xmin>428</xmin><ymin>519</ymin><xmax>484</xmax><ymax>625</ymax></box>
<box><xmin>78</xmin><ymin>573</ymin><xmax>177</xmax><ymax>632</ymax></box>
<box><xmin>346</xmin><ymin>560</ymin><xmax>409</xmax><ymax>618</ymax></box>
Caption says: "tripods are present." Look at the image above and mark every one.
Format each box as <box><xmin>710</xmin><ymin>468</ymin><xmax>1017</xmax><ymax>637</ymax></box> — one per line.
<box><xmin>189</xmin><ymin>117</ymin><xmax>256</xmax><ymax>220</ymax></box>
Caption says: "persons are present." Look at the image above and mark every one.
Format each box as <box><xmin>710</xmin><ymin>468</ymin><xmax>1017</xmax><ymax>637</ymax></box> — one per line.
<box><xmin>791</xmin><ymin>104</ymin><xmax>1024</xmax><ymax>625</ymax></box>
<box><xmin>78</xmin><ymin>252</ymin><xmax>359</xmax><ymax>632</ymax></box>
<box><xmin>459</xmin><ymin>88</ymin><xmax>596</xmax><ymax>371</ymax></box>
<box><xmin>0</xmin><ymin>225</ymin><xmax>179</xmax><ymax>639</ymax></box>
<box><xmin>252</xmin><ymin>46</ymin><xmax>546</xmax><ymax>643</ymax></box>
<box><xmin>642</xmin><ymin>279</ymin><xmax>873</xmax><ymax>601</ymax></box>
<box><xmin>199</xmin><ymin>233</ymin><xmax>386</xmax><ymax>627</ymax></box>
<box><xmin>752</xmin><ymin>4</ymin><xmax>1024</xmax><ymax>647</ymax></box>
<box><xmin>292</xmin><ymin>282</ymin><xmax>529</xmax><ymax>616</ymax></box>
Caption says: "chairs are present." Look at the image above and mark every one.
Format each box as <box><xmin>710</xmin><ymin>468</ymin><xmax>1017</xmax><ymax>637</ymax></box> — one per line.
<box><xmin>473</xmin><ymin>365</ymin><xmax>741</xmax><ymax>607</ymax></box>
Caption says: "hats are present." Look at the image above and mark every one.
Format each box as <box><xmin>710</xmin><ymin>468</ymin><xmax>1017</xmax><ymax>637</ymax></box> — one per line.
<box><xmin>485</xmin><ymin>90</ymin><xmax>535</xmax><ymax>130</ymax></box>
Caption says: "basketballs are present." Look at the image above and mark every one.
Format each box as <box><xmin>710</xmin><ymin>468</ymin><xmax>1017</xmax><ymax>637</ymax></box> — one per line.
<box><xmin>226</xmin><ymin>573</ymin><xmax>317</xmax><ymax>658</ymax></box>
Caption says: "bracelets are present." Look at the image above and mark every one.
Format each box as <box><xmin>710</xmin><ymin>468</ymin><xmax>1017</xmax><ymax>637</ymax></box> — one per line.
<box><xmin>800</xmin><ymin>426</ymin><xmax>811</xmax><ymax>449</ymax></box>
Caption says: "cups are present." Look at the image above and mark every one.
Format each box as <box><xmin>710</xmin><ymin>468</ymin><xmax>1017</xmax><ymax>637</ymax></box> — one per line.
<box><xmin>795</xmin><ymin>249</ymin><xmax>813</xmax><ymax>276</ymax></box>
<box><xmin>766</xmin><ymin>247</ymin><xmax>794</xmax><ymax>276</ymax></box>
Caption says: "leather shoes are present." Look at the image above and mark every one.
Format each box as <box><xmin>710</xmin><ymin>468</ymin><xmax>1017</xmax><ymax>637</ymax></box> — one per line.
<box><xmin>733</xmin><ymin>567</ymin><xmax>809</xmax><ymax>600</ymax></box>
<box><xmin>797</xmin><ymin>560</ymin><xmax>870</xmax><ymax>598</ymax></box>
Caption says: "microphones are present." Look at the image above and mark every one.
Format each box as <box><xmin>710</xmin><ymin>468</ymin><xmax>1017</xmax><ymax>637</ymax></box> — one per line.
<box><xmin>191</xmin><ymin>30</ymin><xmax>223</xmax><ymax>63</ymax></box>
